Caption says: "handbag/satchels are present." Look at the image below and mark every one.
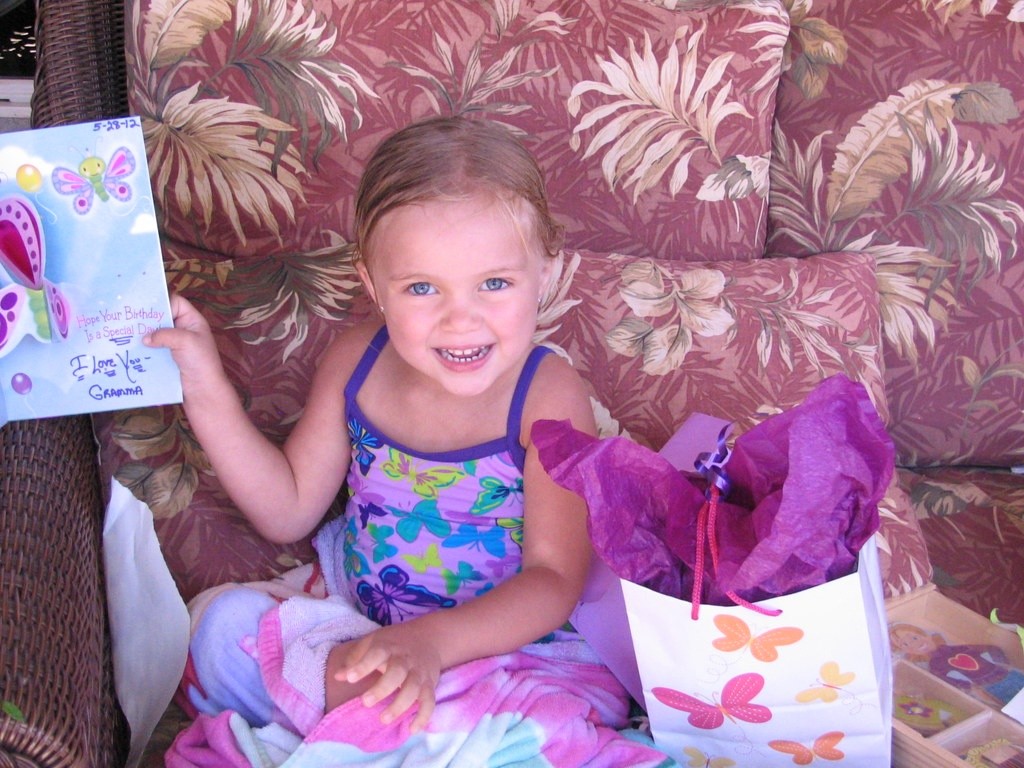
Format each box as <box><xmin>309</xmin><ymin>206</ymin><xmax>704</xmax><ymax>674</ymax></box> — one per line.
<box><xmin>618</xmin><ymin>482</ymin><xmax>894</xmax><ymax>768</ymax></box>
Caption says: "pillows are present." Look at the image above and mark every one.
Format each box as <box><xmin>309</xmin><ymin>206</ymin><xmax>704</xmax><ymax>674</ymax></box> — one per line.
<box><xmin>83</xmin><ymin>1</ymin><xmax>1024</xmax><ymax>606</ymax></box>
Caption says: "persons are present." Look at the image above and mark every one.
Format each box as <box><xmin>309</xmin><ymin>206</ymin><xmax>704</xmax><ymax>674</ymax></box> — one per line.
<box><xmin>144</xmin><ymin>115</ymin><xmax>687</xmax><ymax>768</ymax></box>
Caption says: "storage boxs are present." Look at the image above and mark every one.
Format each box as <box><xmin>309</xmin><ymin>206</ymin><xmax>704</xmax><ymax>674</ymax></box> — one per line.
<box><xmin>882</xmin><ymin>583</ymin><xmax>1024</xmax><ymax>767</ymax></box>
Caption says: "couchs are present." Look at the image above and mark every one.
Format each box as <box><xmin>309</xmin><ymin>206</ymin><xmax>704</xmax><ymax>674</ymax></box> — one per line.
<box><xmin>0</xmin><ymin>0</ymin><xmax>1024</xmax><ymax>768</ymax></box>
